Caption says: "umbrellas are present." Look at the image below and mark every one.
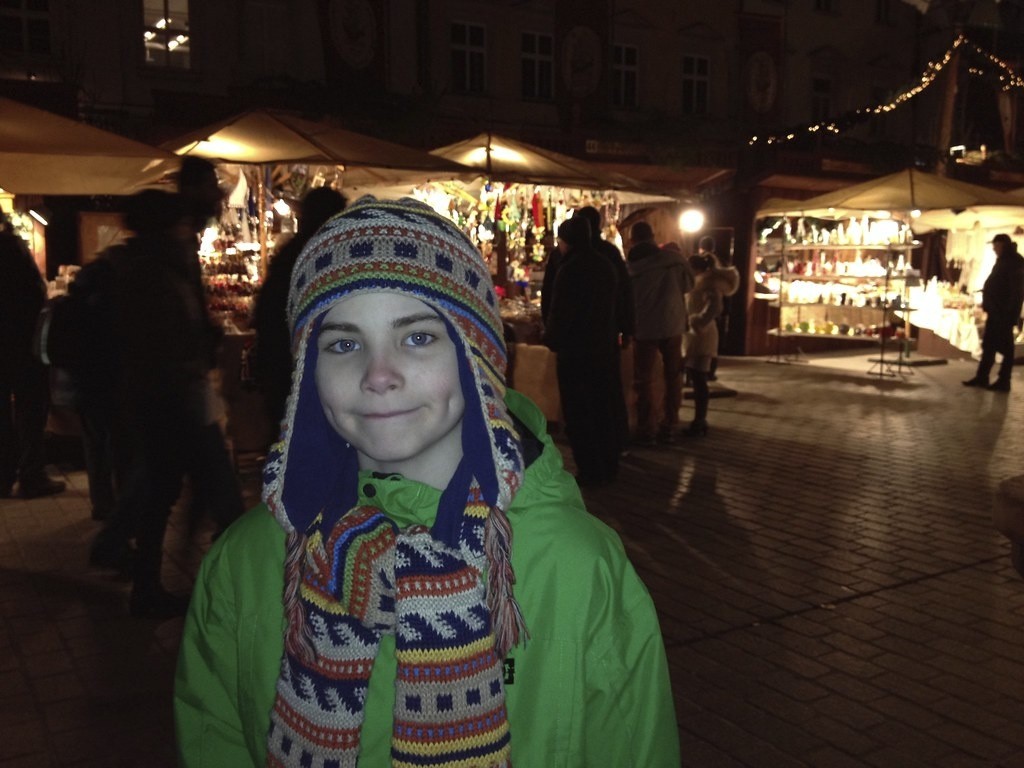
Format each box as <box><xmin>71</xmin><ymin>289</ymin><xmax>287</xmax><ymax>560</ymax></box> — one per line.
<box><xmin>0</xmin><ymin>95</ymin><xmax>1024</xmax><ymax>354</ymax></box>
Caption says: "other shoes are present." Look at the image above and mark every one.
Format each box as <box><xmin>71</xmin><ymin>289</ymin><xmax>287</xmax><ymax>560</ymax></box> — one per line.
<box><xmin>962</xmin><ymin>375</ymin><xmax>989</xmax><ymax>387</ymax></box>
<box><xmin>986</xmin><ymin>378</ymin><xmax>1010</xmax><ymax>391</ymax></box>
<box><xmin>128</xmin><ymin>578</ymin><xmax>190</xmax><ymax>618</ymax></box>
<box><xmin>91</xmin><ymin>497</ymin><xmax>118</xmax><ymax>522</ymax></box>
<box><xmin>16</xmin><ymin>468</ymin><xmax>67</xmax><ymax>495</ymax></box>
<box><xmin>659</xmin><ymin>426</ymin><xmax>676</xmax><ymax>443</ymax></box>
<box><xmin>88</xmin><ymin>528</ymin><xmax>142</xmax><ymax>571</ymax></box>
<box><xmin>629</xmin><ymin>430</ymin><xmax>656</xmax><ymax>447</ymax></box>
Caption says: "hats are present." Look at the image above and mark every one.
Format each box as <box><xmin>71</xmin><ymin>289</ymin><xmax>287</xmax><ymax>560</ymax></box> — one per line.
<box><xmin>986</xmin><ymin>234</ymin><xmax>1011</xmax><ymax>244</ymax></box>
<box><xmin>558</xmin><ymin>217</ymin><xmax>591</xmax><ymax>248</ymax></box>
<box><xmin>263</xmin><ymin>192</ymin><xmax>531</xmax><ymax>674</ymax></box>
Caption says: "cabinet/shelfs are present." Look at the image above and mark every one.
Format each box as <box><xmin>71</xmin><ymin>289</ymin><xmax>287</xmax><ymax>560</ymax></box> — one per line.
<box><xmin>766</xmin><ymin>241</ymin><xmax>922</xmax><ymax>378</ymax></box>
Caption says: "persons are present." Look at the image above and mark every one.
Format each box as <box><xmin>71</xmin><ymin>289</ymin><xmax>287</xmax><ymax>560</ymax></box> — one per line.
<box><xmin>0</xmin><ymin>214</ymin><xmax>67</xmax><ymax>500</ymax></box>
<box><xmin>528</xmin><ymin>205</ymin><xmax>637</xmax><ymax>488</ymax></box>
<box><xmin>626</xmin><ymin>221</ymin><xmax>695</xmax><ymax>444</ymax></box>
<box><xmin>961</xmin><ymin>234</ymin><xmax>1024</xmax><ymax>393</ymax></box>
<box><xmin>53</xmin><ymin>155</ymin><xmax>245</xmax><ymax>618</ymax></box>
<box><xmin>173</xmin><ymin>195</ymin><xmax>684</xmax><ymax>768</ymax></box>
<box><xmin>251</xmin><ymin>186</ymin><xmax>347</xmax><ymax>482</ymax></box>
<box><xmin>680</xmin><ymin>234</ymin><xmax>723</xmax><ymax>436</ymax></box>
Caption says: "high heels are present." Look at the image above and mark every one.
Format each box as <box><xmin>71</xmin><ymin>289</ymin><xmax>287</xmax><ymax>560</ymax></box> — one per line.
<box><xmin>682</xmin><ymin>418</ymin><xmax>708</xmax><ymax>437</ymax></box>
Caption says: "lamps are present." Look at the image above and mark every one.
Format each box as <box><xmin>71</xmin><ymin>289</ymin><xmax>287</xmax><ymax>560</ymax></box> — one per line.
<box><xmin>27</xmin><ymin>203</ymin><xmax>55</xmax><ymax>226</ymax></box>
